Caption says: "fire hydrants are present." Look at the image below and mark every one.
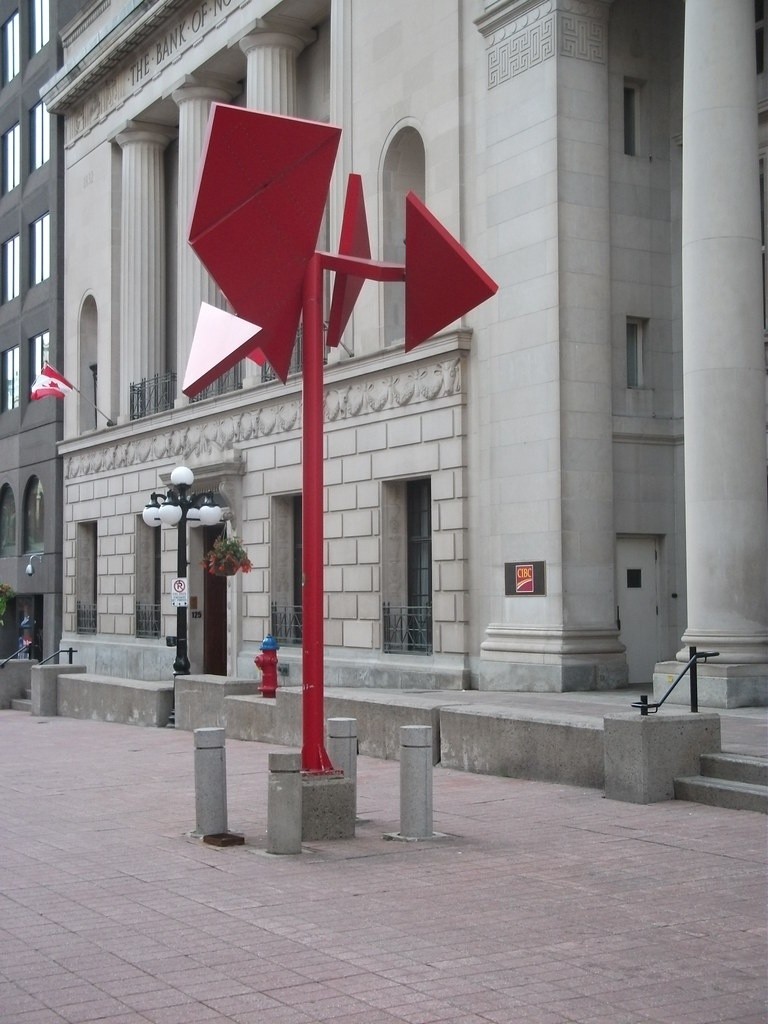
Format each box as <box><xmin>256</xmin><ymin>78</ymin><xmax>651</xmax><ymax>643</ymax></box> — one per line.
<box><xmin>254</xmin><ymin>633</ymin><xmax>282</xmax><ymax>699</ymax></box>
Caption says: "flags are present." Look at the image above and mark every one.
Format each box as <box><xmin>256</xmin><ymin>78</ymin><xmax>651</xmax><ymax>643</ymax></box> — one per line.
<box><xmin>30</xmin><ymin>365</ymin><xmax>75</xmax><ymax>401</ymax></box>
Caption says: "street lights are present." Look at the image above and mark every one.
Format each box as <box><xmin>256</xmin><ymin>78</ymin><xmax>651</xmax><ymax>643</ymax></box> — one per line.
<box><xmin>142</xmin><ymin>466</ymin><xmax>224</xmax><ymax>678</ymax></box>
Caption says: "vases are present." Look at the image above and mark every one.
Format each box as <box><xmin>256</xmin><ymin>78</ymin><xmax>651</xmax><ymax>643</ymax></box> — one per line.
<box><xmin>215</xmin><ymin>562</ymin><xmax>240</xmax><ymax>577</ymax></box>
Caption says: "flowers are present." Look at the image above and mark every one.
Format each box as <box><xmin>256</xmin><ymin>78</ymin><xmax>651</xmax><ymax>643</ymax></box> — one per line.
<box><xmin>197</xmin><ymin>532</ymin><xmax>254</xmax><ymax>576</ymax></box>
